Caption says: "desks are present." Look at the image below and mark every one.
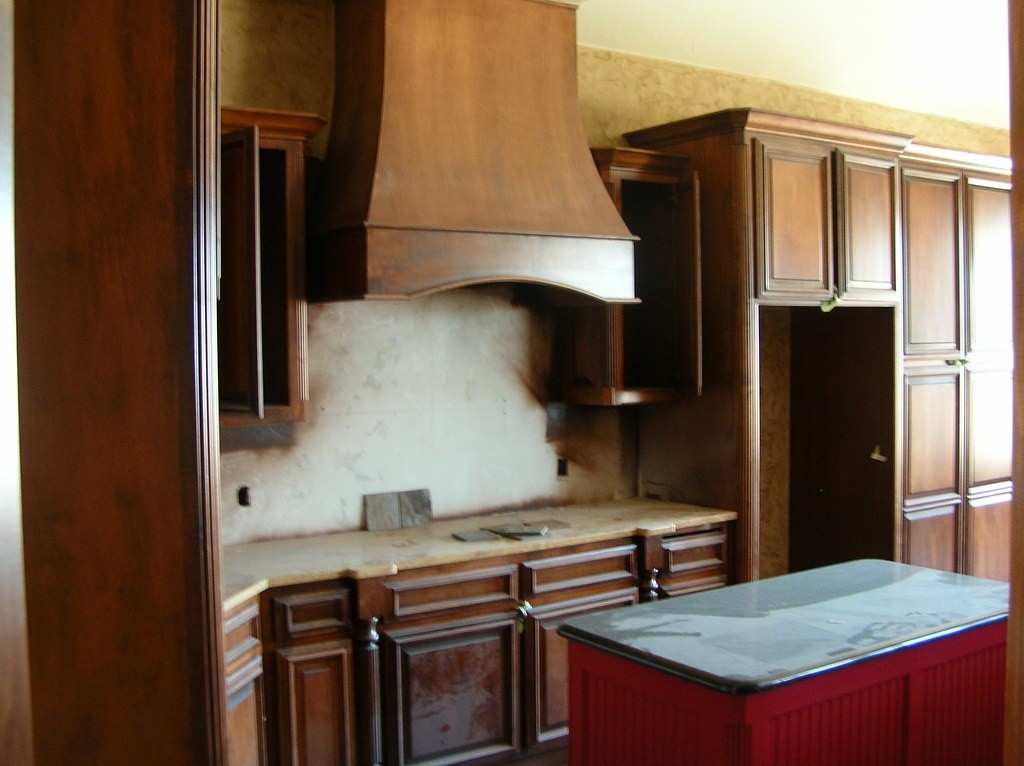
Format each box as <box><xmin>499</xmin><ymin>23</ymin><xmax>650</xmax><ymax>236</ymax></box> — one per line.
<box><xmin>557</xmin><ymin>557</ymin><xmax>1011</xmax><ymax>766</ymax></box>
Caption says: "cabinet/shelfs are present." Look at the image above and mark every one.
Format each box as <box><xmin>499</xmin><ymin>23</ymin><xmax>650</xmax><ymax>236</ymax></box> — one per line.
<box><xmin>518</xmin><ymin>521</ymin><xmax>732</xmax><ymax>766</ymax></box>
<box><xmin>550</xmin><ymin>146</ymin><xmax>703</xmax><ymax>407</ymax></box>
<box><xmin>218</xmin><ymin>105</ymin><xmax>329</xmax><ymax>430</ymax></box>
<box><xmin>260</xmin><ymin>554</ymin><xmax>519</xmax><ymax>766</ymax></box>
<box><xmin>621</xmin><ymin>107</ymin><xmax>914</xmax><ymax>307</ymax></box>
<box><xmin>788</xmin><ymin>144</ymin><xmax>1012</xmax><ymax>585</ymax></box>
